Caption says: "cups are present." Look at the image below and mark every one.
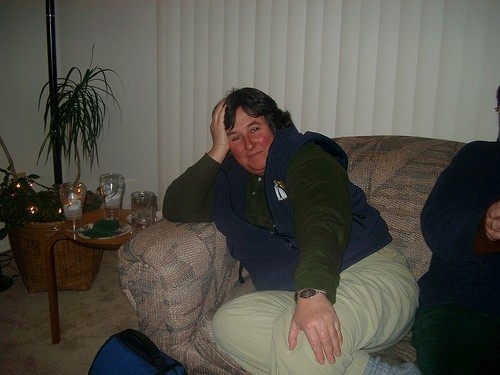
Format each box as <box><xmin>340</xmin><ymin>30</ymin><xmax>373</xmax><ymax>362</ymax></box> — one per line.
<box><xmin>99</xmin><ymin>173</ymin><xmax>125</xmax><ymax>221</ymax></box>
<box><xmin>59</xmin><ymin>181</ymin><xmax>85</xmax><ymax>230</ymax></box>
<box><xmin>131</xmin><ymin>191</ymin><xmax>157</xmax><ymax>235</ymax></box>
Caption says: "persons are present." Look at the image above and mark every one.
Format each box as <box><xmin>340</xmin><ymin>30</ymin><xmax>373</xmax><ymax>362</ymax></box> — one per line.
<box><xmin>162</xmin><ymin>87</ymin><xmax>420</xmax><ymax>375</ymax></box>
<box><xmin>415</xmin><ymin>86</ymin><xmax>500</xmax><ymax>375</ymax></box>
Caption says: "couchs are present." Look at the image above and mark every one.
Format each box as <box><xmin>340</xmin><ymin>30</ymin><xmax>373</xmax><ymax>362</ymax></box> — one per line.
<box><xmin>119</xmin><ymin>135</ymin><xmax>468</xmax><ymax>375</ymax></box>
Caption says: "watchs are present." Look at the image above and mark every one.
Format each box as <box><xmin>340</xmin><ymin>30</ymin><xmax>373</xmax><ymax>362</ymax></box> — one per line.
<box><xmin>297</xmin><ymin>288</ymin><xmax>326</xmax><ymax>299</ymax></box>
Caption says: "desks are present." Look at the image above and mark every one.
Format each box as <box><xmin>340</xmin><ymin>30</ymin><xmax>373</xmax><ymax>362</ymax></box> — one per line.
<box><xmin>45</xmin><ymin>208</ymin><xmax>156</xmax><ymax>344</ymax></box>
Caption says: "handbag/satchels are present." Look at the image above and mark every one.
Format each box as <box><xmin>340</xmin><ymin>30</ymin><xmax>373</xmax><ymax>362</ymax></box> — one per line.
<box><xmin>88</xmin><ymin>328</ymin><xmax>187</xmax><ymax>375</ymax></box>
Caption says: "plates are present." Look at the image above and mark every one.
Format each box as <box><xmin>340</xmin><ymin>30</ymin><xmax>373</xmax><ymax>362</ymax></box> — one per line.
<box><xmin>78</xmin><ymin>221</ymin><xmax>132</xmax><ymax>239</ymax></box>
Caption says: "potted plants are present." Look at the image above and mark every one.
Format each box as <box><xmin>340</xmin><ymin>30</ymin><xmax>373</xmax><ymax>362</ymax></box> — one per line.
<box><xmin>0</xmin><ymin>40</ymin><xmax>103</xmax><ymax>292</ymax></box>
